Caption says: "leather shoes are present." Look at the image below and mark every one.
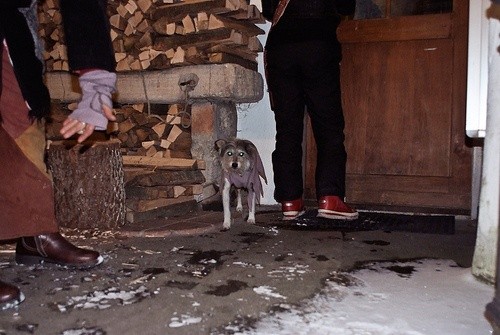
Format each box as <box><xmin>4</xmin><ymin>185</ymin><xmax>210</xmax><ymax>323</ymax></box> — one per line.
<box><xmin>15</xmin><ymin>232</ymin><xmax>104</xmax><ymax>270</ymax></box>
<box><xmin>0</xmin><ymin>280</ymin><xmax>25</xmax><ymax>310</ymax></box>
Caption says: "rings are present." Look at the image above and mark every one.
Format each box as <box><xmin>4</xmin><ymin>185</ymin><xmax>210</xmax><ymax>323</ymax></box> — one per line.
<box><xmin>81</xmin><ymin>122</ymin><xmax>86</xmax><ymax>127</ymax></box>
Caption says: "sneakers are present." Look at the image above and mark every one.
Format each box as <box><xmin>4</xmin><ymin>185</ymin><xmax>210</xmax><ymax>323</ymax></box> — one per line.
<box><xmin>316</xmin><ymin>195</ymin><xmax>359</xmax><ymax>220</ymax></box>
<box><xmin>281</xmin><ymin>198</ymin><xmax>307</xmax><ymax>220</ymax></box>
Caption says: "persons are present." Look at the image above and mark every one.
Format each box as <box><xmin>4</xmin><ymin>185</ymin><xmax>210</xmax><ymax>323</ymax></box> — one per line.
<box><xmin>261</xmin><ymin>0</ymin><xmax>359</xmax><ymax>221</ymax></box>
<box><xmin>0</xmin><ymin>0</ymin><xmax>117</xmax><ymax>311</ymax></box>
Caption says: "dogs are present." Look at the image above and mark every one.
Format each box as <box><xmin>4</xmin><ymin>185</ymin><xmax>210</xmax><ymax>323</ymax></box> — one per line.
<box><xmin>214</xmin><ymin>138</ymin><xmax>268</xmax><ymax>231</ymax></box>
